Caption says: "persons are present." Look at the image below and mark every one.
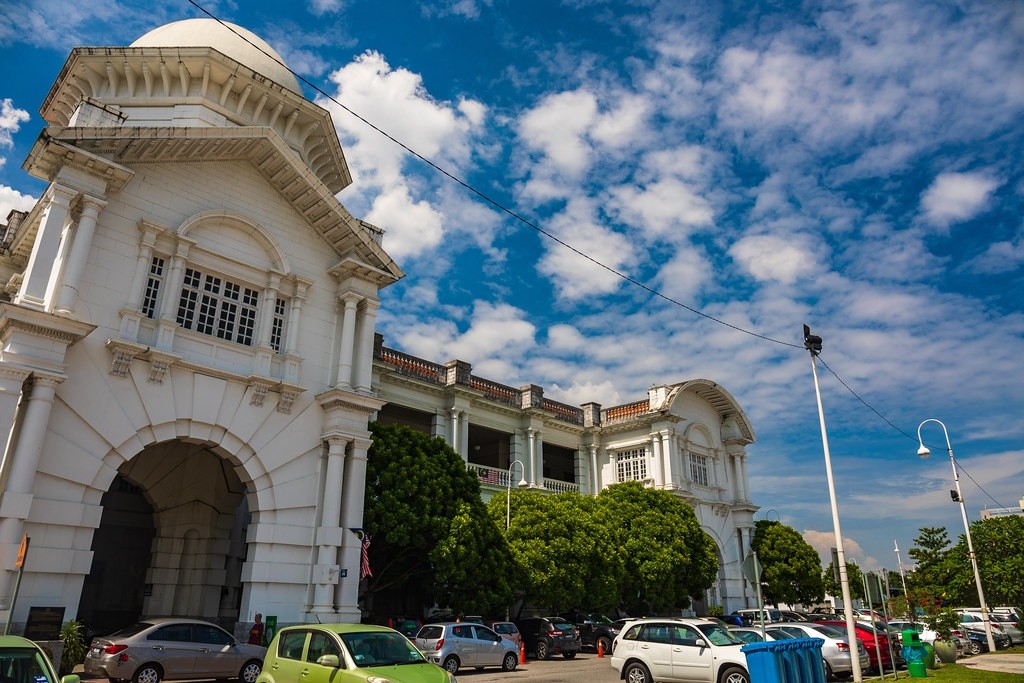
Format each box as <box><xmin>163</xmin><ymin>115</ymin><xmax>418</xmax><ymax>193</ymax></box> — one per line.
<box><xmin>248</xmin><ymin>613</ymin><xmax>264</xmax><ymax>644</ymax></box>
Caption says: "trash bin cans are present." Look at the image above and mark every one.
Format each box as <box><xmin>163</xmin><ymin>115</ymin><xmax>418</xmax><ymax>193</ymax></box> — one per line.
<box><xmin>901</xmin><ymin>630</ymin><xmax>928</xmax><ymax>678</ymax></box>
<box><xmin>738</xmin><ymin>637</ymin><xmax>828</xmax><ymax>683</ymax></box>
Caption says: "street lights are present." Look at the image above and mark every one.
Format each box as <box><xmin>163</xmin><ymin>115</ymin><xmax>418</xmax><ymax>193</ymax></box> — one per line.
<box><xmin>893</xmin><ymin>540</ymin><xmax>909</xmax><ymax>605</ymax></box>
<box><xmin>915</xmin><ymin>418</ymin><xmax>995</xmax><ymax>652</ymax></box>
<box><xmin>505</xmin><ymin>459</ymin><xmax>528</xmax><ymax>530</ymax></box>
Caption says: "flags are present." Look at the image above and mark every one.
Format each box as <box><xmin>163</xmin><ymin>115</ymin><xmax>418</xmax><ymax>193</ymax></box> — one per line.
<box><xmin>361</xmin><ymin>534</ymin><xmax>372</xmax><ymax>582</ymax></box>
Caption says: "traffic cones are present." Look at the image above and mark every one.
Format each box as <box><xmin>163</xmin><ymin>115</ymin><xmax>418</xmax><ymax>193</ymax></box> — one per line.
<box><xmin>598</xmin><ymin>639</ymin><xmax>605</xmax><ymax>658</ymax></box>
<box><xmin>518</xmin><ymin>641</ymin><xmax>529</xmax><ymax>665</ymax></box>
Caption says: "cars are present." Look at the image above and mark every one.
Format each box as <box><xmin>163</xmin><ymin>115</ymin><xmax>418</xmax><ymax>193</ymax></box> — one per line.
<box><xmin>0</xmin><ymin>633</ymin><xmax>82</xmax><ymax>682</ymax></box>
<box><xmin>515</xmin><ymin>616</ymin><xmax>582</xmax><ymax>661</ymax></box>
<box><xmin>415</xmin><ymin>622</ymin><xmax>520</xmax><ymax>676</ymax></box>
<box><xmin>564</xmin><ymin>612</ymin><xmax>622</xmax><ymax>655</ymax></box>
<box><xmin>483</xmin><ymin>621</ymin><xmax>521</xmax><ymax>647</ymax></box>
<box><xmin>815</xmin><ymin>620</ymin><xmax>900</xmax><ymax>675</ymax></box>
<box><xmin>83</xmin><ymin>617</ymin><xmax>268</xmax><ymax>683</ymax></box>
<box><xmin>256</xmin><ymin>622</ymin><xmax>458</xmax><ymax>683</ymax></box>
<box><xmin>717</xmin><ymin>606</ymin><xmax>1024</xmax><ymax>655</ymax></box>
<box><xmin>761</xmin><ymin>623</ymin><xmax>870</xmax><ymax>678</ymax></box>
<box><xmin>392</xmin><ymin>618</ymin><xmax>424</xmax><ymax>643</ymax></box>
<box><xmin>726</xmin><ymin>627</ymin><xmax>795</xmax><ymax>644</ymax></box>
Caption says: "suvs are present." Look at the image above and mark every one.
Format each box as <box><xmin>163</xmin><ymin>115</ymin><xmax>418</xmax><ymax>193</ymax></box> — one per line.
<box><xmin>609</xmin><ymin>616</ymin><xmax>754</xmax><ymax>683</ymax></box>
